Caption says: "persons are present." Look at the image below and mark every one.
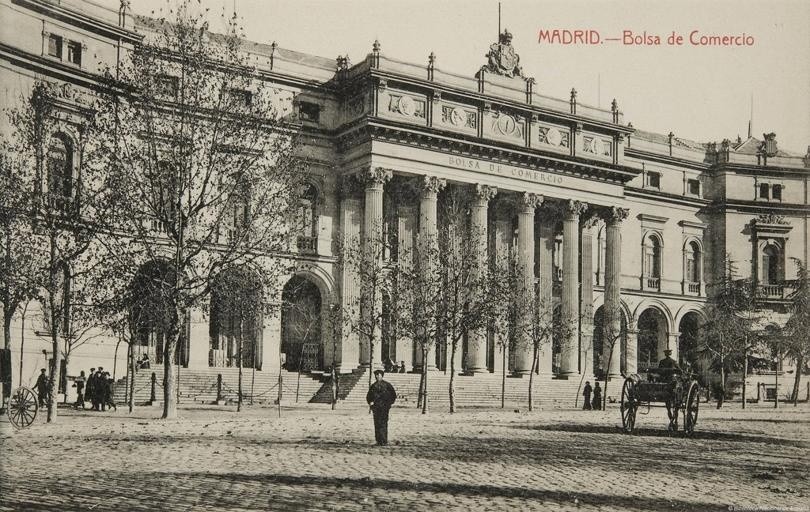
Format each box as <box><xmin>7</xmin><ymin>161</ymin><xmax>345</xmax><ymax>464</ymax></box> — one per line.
<box><xmin>398</xmin><ymin>360</ymin><xmax>405</xmax><ymax>373</ymax></box>
<box><xmin>366</xmin><ymin>369</ymin><xmax>397</xmax><ymax>446</ymax></box>
<box><xmin>72</xmin><ymin>367</ymin><xmax>119</xmax><ymax>410</ymax></box>
<box><xmin>32</xmin><ymin>368</ymin><xmax>49</xmax><ymax>406</ymax></box>
<box><xmin>582</xmin><ymin>380</ymin><xmax>592</xmax><ymax>410</ymax></box>
<box><xmin>591</xmin><ymin>381</ymin><xmax>602</xmax><ymax>411</ymax></box>
<box><xmin>141</xmin><ymin>353</ymin><xmax>151</xmax><ymax>369</ymax></box>
<box><xmin>659</xmin><ymin>349</ymin><xmax>682</xmax><ymax>383</ymax></box>
<box><xmin>489</xmin><ymin>30</ymin><xmax>517</xmax><ymax>78</ymax></box>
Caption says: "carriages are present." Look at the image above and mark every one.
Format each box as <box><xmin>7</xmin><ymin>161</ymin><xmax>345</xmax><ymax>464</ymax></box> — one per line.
<box><xmin>621</xmin><ymin>356</ymin><xmax>701</xmax><ymax>438</ymax></box>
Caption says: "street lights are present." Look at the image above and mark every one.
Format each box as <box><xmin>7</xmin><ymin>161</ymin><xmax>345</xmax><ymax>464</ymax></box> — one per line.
<box><xmin>773</xmin><ymin>355</ymin><xmax>779</xmax><ymax>409</ymax></box>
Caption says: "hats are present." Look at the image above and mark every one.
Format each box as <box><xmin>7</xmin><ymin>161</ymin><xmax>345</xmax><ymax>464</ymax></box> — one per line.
<box><xmin>663</xmin><ymin>350</ymin><xmax>672</xmax><ymax>355</ymax></box>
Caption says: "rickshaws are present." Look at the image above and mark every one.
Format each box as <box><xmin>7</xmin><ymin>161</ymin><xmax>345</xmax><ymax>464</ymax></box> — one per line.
<box><xmin>1</xmin><ymin>349</ymin><xmax>38</xmax><ymax>431</ymax></box>
